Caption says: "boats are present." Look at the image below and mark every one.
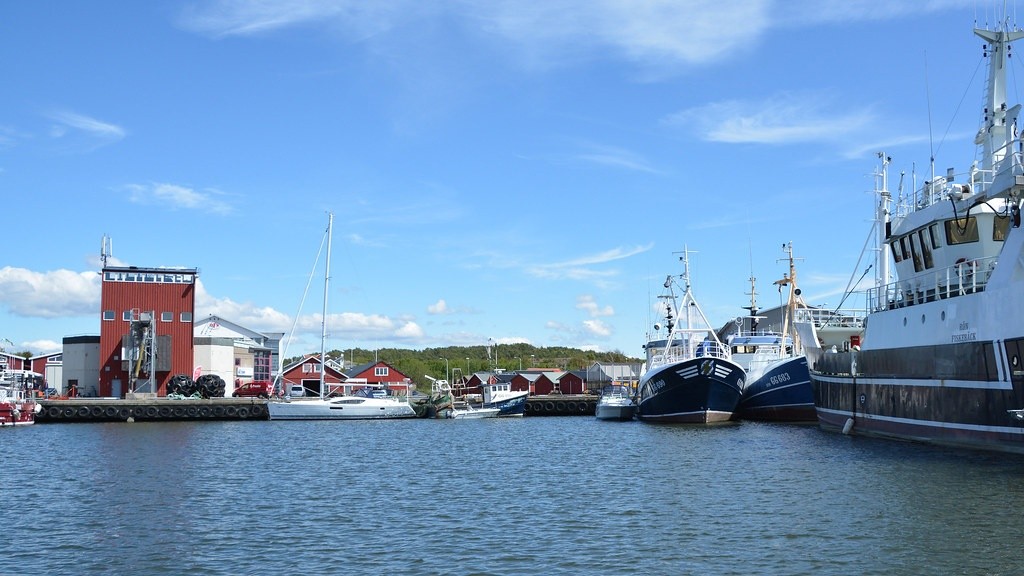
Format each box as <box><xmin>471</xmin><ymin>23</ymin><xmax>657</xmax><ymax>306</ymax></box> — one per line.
<box><xmin>595</xmin><ymin>391</ymin><xmax>637</xmax><ymax>420</ymax></box>
<box><xmin>0</xmin><ymin>355</ymin><xmax>42</xmax><ymax>427</ymax></box>
<box><xmin>726</xmin><ymin>240</ymin><xmax>818</xmax><ymax>422</ymax></box>
<box><xmin>452</xmin><ymin>384</ymin><xmax>528</xmax><ymax>417</ymax></box>
<box><xmin>436</xmin><ymin>408</ymin><xmax>501</xmax><ymax>419</ymax></box>
<box><xmin>792</xmin><ymin>14</ymin><xmax>1024</xmax><ymax>457</ymax></box>
<box><xmin>632</xmin><ymin>244</ymin><xmax>748</xmax><ymax>424</ymax></box>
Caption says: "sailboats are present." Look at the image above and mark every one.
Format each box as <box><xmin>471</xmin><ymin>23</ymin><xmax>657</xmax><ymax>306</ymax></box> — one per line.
<box><xmin>267</xmin><ymin>213</ymin><xmax>417</xmax><ymax>419</ymax></box>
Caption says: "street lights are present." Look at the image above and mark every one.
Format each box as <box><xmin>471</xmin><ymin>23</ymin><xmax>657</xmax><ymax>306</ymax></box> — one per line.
<box><xmin>514</xmin><ymin>357</ymin><xmax>521</xmax><ymax>371</ymax></box>
<box><xmin>466</xmin><ymin>358</ymin><xmax>469</xmax><ymax>394</ymax></box>
<box><xmin>531</xmin><ymin>355</ymin><xmax>534</xmax><ymax>368</ymax></box>
<box><xmin>440</xmin><ymin>358</ymin><xmax>448</xmax><ymax>381</ymax></box>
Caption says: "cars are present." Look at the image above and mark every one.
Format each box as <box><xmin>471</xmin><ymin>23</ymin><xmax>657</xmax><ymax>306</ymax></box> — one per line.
<box><xmin>232</xmin><ymin>382</ymin><xmax>274</xmax><ymax>399</ymax></box>
<box><xmin>44</xmin><ymin>388</ymin><xmax>57</xmax><ymax>395</ymax></box>
<box><xmin>291</xmin><ymin>385</ymin><xmax>306</xmax><ymax>397</ymax></box>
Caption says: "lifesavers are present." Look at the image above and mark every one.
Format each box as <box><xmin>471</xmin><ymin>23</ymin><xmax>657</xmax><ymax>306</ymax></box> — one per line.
<box><xmin>199</xmin><ymin>406</ymin><xmax>211</xmax><ymax>418</ymax></box>
<box><xmin>105</xmin><ymin>406</ymin><xmax>117</xmax><ymax>417</ymax></box>
<box><xmin>251</xmin><ymin>405</ymin><xmax>262</xmax><ymax>418</ymax></box>
<box><xmin>146</xmin><ymin>406</ymin><xmax>158</xmax><ymax>417</ymax></box>
<box><xmin>78</xmin><ymin>406</ymin><xmax>91</xmax><ymax>418</ymax></box>
<box><xmin>63</xmin><ymin>407</ymin><xmax>76</xmax><ymax>418</ymax></box>
<box><xmin>49</xmin><ymin>407</ymin><xmax>61</xmax><ymax>418</ymax></box>
<box><xmin>238</xmin><ymin>407</ymin><xmax>250</xmax><ymax>419</ymax></box>
<box><xmin>173</xmin><ymin>406</ymin><xmax>185</xmax><ymax>417</ymax></box>
<box><xmin>955</xmin><ymin>257</ymin><xmax>973</xmax><ymax>280</ymax></box>
<box><xmin>36</xmin><ymin>407</ymin><xmax>47</xmax><ymax>418</ymax></box>
<box><xmin>818</xmin><ymin>338</ymin><xmax>824</xmax><ymax>349</ymax></box>
<box><xmin>226</xmin><ymin>405</ymin><xmax>237</xmax><ymax>417</ymax></box>
<box><xmin>160</xmin><ymin>406</ymin><xmax>171</xmax><ymax>418</ymax></box>
<box><xmin>133</xmin><ymin>406</ymin><xmax>145</xmax><ymax>418</ymax></box>
<box><xmin>212</xmin><ymin>405</ymin><xmax>225</xmax><ymax>418</ymax></box>
<box><xmin>119</xmin><ymin>407</ymin><xmax>130</xmax><ymax>420</ymax></box>
<box><xmin>187</xmin><ymin>406</ymin><xmax>198</xmax><ymax>417</ymax></box>
<box><xmin>92</xmin><ymin>406</ymin><xmax>104</xmax><ymax>418</ymax></box>
